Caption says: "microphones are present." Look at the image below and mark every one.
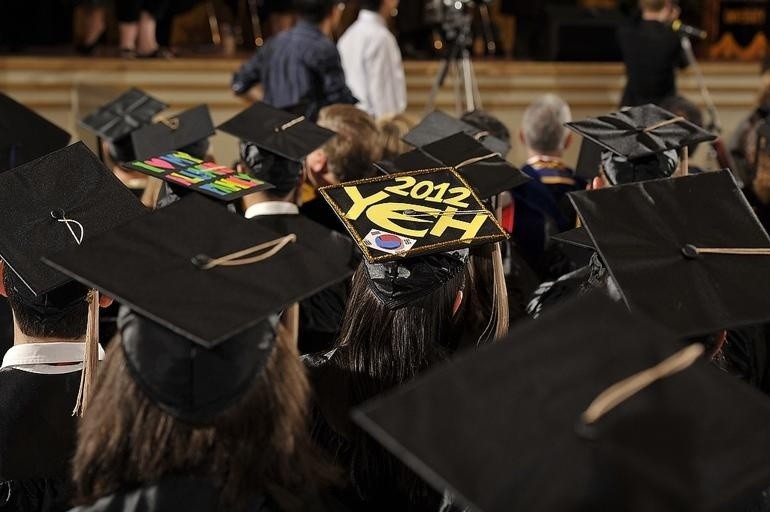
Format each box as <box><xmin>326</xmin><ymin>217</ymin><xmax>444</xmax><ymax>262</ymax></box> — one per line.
<box><xmin>671</xmin><ymin>19</ymin><xmax>708</xmax><ymax>40</ymax></box>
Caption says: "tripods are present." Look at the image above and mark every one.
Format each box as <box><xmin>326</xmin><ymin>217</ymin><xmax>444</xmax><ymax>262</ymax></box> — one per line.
<box><xmin>421</xmin><ymin>49</ymin><xmax>483</xmax><ymax>121</ymax></box>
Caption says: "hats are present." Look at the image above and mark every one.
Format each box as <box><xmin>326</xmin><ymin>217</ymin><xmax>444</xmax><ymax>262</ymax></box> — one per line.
<box><xmin>0</xmin><ymin>87</ymin><xmax>769</xmax><ymax>510</ymax></box>
